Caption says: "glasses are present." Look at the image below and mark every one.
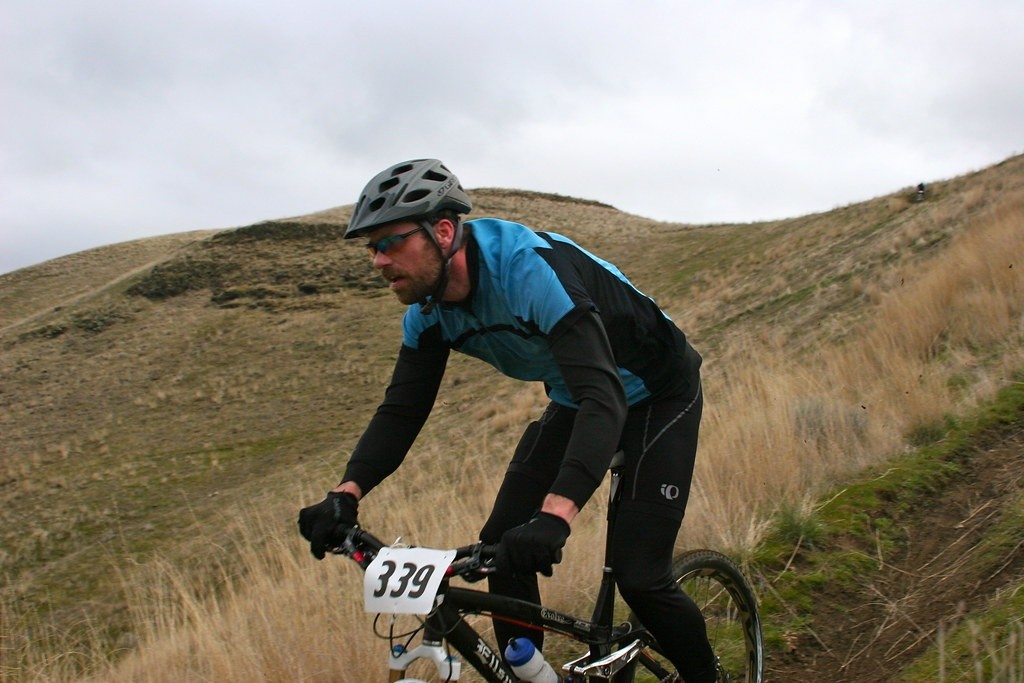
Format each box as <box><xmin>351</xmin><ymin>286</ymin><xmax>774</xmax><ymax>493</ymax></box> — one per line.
<box><xmin>364</xmin><ymin>227</ymin><xmax>423</xmax><ymax>256</ymax></box>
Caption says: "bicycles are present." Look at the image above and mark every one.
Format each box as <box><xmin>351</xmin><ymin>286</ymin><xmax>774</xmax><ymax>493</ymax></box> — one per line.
<box><xmin>317</xmin><ymin>512</ymin><xmax>766</xmax><ymax>683</ymax></box>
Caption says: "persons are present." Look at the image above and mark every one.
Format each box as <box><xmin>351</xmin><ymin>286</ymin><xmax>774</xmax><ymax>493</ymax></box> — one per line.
<box><xmin>298</xmin><ymin>157</ymin><xmax>720</xmax><ymax>683</ymax></box>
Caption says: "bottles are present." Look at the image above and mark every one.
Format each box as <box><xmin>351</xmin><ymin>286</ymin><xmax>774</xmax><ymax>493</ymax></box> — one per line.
<box><xmin>504</xmin><ymin>637</ymin><xmax>558</xmax><ymax>683</ymax></box>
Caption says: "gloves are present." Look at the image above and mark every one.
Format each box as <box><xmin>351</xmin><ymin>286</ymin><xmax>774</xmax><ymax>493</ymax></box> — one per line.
<box><xmin>498</xmin><ymin>512</ymin><xmax>571</xmax><ymax>578</ymax></box>
<box><xmin>297</xmin><ymin>492</ymin><xmax>360</xmax><ymax>561</ymax></box>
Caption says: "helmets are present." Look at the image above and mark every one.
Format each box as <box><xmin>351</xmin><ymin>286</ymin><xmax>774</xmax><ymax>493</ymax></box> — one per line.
<box><xmin>341</xmin><ymin>158</ymin><xmax>473</xmax><ymax>240</ymax></box>
